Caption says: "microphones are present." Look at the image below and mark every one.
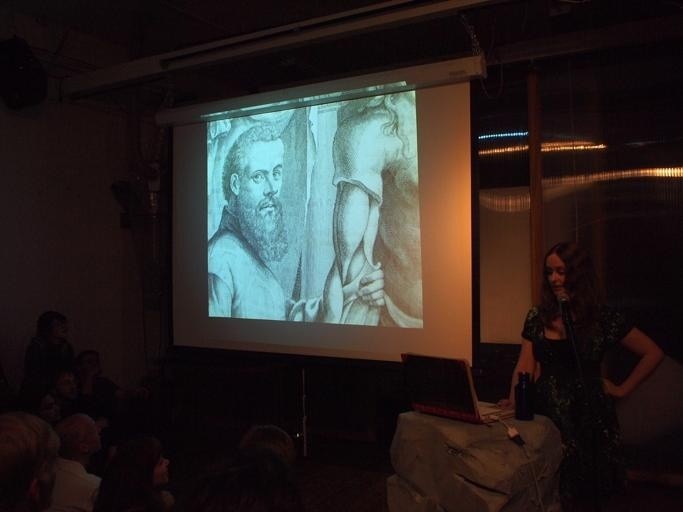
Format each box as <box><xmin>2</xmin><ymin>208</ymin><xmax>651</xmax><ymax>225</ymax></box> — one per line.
<box><xmin>557</xmin><ymin>293</ymin><xmax>575</xmax><ymax>330</ymax></box>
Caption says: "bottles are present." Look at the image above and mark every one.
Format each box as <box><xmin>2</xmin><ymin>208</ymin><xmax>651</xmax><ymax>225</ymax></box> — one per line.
<box><xmin>512</xmin><ymin>371</ymin><xmax>536</xmax><ymax>420</ymax></box>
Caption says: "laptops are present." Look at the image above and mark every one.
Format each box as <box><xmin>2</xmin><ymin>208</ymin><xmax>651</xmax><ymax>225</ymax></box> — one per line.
<box><xmin>400</xmin><ymin>352</ymin><xmax>516</xmax><ymax>425</ymax></box>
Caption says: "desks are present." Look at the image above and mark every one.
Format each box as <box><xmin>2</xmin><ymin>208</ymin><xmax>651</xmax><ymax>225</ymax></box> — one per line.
<box><xmin>384</xmin><ymin>408</ymin><xmax>564</xmax><ymax>512</ymax></box>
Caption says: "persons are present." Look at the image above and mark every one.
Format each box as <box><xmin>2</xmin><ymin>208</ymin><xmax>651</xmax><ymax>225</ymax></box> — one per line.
<box><xmin>208</xmin><ymin>124</ymin><xmax>384</xmax><ymax>321</ymax></box>
<box><xmin>509</xmin><ymin>241</ymin><xmax>665</xmax><ymax>512</ymax></box>
<box><xmin>320</xmin><ymin>80</ymin><xmax>423</xmax><ymax>329</ymax></box>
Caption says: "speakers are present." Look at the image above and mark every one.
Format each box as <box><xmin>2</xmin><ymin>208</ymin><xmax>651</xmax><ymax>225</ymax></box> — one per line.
<box><xmin>0</xmin><ymin>35</ymin><xmax>48</xmax><ymax>112</ymax></box>
